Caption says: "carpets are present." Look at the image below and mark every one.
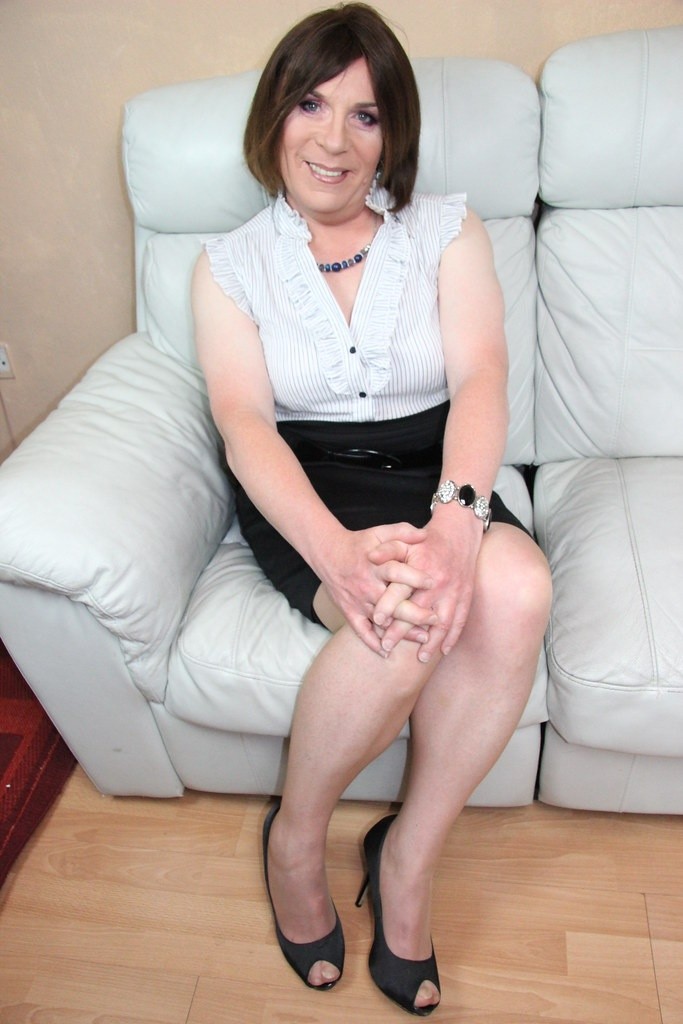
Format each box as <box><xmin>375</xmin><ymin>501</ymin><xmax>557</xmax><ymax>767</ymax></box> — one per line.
<box><xmin>0</xmin><ymin>644</ymin><xmax>76</xmax><ymax>885</ymax></box>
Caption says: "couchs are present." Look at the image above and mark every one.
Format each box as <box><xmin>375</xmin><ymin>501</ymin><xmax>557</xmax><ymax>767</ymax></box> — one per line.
<box><xmin>0</xmin><ymin>26</ymin><xmax>682</xmax><ymax>817</ymax></box>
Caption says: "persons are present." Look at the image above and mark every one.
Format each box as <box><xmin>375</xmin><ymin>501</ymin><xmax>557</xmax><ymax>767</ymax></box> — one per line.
<box><xmin>191</xmin><ymin>1</ymin><xmax>557</xmax><ymax>1016</ymax></box>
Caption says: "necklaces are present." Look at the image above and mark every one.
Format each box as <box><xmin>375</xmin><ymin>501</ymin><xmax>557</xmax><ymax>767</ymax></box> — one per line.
<box><xmin>316</xmin><ymin>241</ymin><xmax>373</xmax><ymax>272</ymax></box>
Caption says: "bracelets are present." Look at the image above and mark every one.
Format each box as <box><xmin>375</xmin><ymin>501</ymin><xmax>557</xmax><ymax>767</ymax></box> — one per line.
<box><xmin>428</xmin><ymin>482</ymin><xmax>489</xmax><ymax>532</ymax></box>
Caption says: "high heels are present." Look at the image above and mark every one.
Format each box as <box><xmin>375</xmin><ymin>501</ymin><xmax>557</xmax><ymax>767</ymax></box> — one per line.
<box><xmin>354</xmin><ymin>813</ymin><xmax>441</xmax><ymax>1016</ymax></box>
<box><xmin>262</xmin><ymin>805</ymin><xmax>345</xmax><ymax>991</ymax></box>
